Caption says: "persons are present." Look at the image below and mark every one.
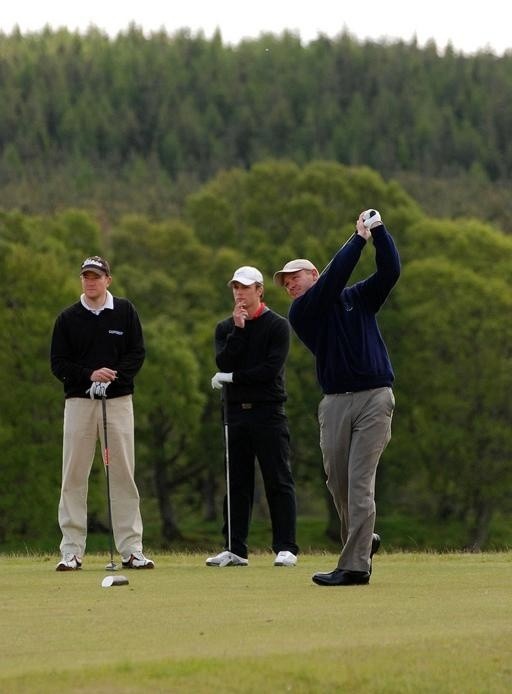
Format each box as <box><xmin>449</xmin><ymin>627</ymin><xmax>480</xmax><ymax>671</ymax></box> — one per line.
<box><xmin>47</xmin><ymin>256</ymin><xmax>158</xmax><ymax>569</ymax></box>
<box><xmin>205</xmin><ymin>267</ymin><xmax>298</xmax><ymax>568</ymax></box>
<box><xmin>272</xmin><ymin>211</ymin><xmax>401</xmax><ymax>588</ymax></box>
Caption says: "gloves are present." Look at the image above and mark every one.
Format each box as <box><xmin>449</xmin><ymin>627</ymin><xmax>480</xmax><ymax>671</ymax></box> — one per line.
<box><xmin>212</xmin><ymin>372</ymin><xmax>234</xmax><ymax>390</ymax></box>
<box><xmin>90</xmin><ymin>380</ymin><xmax>111</xmax><ymax>400</ymax></box>
<box><xmin>362</xmin><ymin>209</ymin><xmax>382</xmax><ymax>231</ymax></box>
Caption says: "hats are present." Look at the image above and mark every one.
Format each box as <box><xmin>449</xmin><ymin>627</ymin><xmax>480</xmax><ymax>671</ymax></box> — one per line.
<box><xmin>227</xmin><ymin>266</ymin><xmax>263</xmax><ymax>287</ymax></box>
<box><xmin>273</xmin><ymin>259</ymin><xmax>319</xmax><ymax>288</ymax></box>
<box><xmin>79</xmin><ymin>255</ymin><xmax>110</xmax><ymax>277</ymax></box>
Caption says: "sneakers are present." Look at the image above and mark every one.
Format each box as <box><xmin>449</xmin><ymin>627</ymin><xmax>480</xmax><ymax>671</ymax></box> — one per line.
<box><xmin>206</xmin><ymin>550</ymin><xmax>248</xmax><ymax>566</ymax></box>
<box><xmin>274</xmin><ymin>550</ymin><xmax>297</xmax><ymax>566</ymax></box>
<box><xmin>56</xmin><ymin>553</ymin><xmax>82</xmax><ymax>570</ymax></box>
<box><xmin>122</xmin><ymin>551</ymin><xmax>154</xmax><ymax>569</ymax></box>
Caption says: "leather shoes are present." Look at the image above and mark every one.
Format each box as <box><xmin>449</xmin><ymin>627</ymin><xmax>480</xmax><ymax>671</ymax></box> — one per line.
<box><xmin>369</xmin><ymin>533</ymin><xmax>380</xmax><ymax>575</ymax></box>
<box><xmin>313</xmin><ymin>568</ymin><xmax>370</xmax><ymax>585</ymax></box>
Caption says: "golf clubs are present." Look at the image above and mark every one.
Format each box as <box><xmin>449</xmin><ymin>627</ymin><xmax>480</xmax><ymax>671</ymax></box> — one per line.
<box><xmin>102</xmin><ymin>396</ymin><xmax>123</xmax><ymax>571</ymax></box>
<box><xmin>219</xmin><ymin>382</ymin><xmax>234</xmax><ymax>567</ymax></box>
